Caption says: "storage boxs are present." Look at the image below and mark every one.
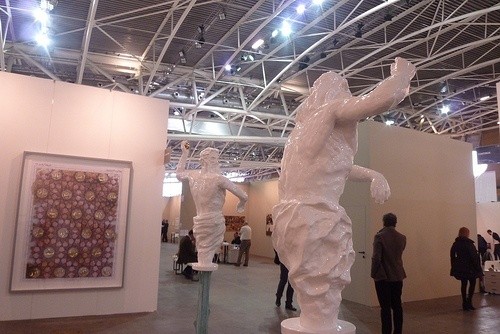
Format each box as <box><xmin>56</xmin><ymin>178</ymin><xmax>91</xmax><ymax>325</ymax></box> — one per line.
<box><xmin>483</xmin><ymin>260</ymin><xmax>500</xmax><ymax>295</ymax></box>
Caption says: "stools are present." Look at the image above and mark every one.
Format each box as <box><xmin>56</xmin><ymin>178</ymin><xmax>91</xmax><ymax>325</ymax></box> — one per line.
<box><xmin>172</xmin><ymin>255</ymin><xmax>184</xmax><ymax>275</ymax></box>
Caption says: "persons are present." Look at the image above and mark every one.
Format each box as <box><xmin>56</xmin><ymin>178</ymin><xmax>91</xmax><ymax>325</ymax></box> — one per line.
<box><xmin>234</xmin><ymin>220</ymin><xmax>252</xmax><ymax>266</ymax></box>
<box><xmin>477</xmin><ymin>230</ymin><xmax>500</xmax><ymax>265</ymax></box>
<box><xmin>177</xmin><ymin>230</ymin><xmax>199</xmax><ymax>280</ymax></box>
<box><xmin>160</xmin><ymin>219</ymin><xmax>169</xmax><ymax>242</ymax></box>
<box><xmin>231</xmin><ymin>232</ymin><xmax>241</xmax><ymax>245</ymax></box>
<box><xmin>450</xmin><ymin>227</ymin><xmax>483</xmax><ymax>310</ymax></box>
<box><xmin>272</xmin><ymin>58</ymin><xmax>417</xmax><ymax>330</ymax></box>
<box><xmin>273</xmin><ymin>249</ymin><xmax>297</xmax><ymax>312</ymax></box>
<box><xmin>371</xmin><ymin>213</ymin><xmax>407</xmax><ymax>334</ymax></box>
<box><xmin>175</xmin><ymin>141</ymin><xmax>248</xmax><ymax>265</ymax></box>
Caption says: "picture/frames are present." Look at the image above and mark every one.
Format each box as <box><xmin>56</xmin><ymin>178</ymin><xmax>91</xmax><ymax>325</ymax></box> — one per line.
<box><xmin>9</xmin><ymin>150</ymin><xmax>133</xmax><ymax>293</ymax></box>
<box><xmin>266</xmin><ymin>215</ymin><xmax>273</xmax><ymax>237</ymax></box>
<box><xmin>225</xmin><ymin>215</ymin><xmax>247</xmax><ymax>232</ymax></box>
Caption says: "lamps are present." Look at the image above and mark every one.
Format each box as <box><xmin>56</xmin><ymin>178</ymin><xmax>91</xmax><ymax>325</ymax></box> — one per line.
<box><xmin>35</xmin><ymin>0</ymin><xmax>363</xmax><ymax>84</ymax></box>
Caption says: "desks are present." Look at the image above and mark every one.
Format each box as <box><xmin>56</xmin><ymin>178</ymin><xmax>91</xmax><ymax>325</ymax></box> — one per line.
<box><xmin>213</xmin><ymin>242</ymin><xmax>244</xmax><ymax>265</ymax></box>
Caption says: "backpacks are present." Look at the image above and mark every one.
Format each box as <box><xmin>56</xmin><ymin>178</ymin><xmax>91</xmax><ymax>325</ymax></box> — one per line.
<box><xmin>450</xmin><ymin>258</ymin><xmax>465</xmax><ymax>280</ymax></box>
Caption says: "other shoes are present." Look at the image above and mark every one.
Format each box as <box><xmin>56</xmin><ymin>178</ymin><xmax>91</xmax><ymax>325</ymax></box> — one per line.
<box><xmin>285</xmin><ymin>302</ymin><xmax>297</xmax><ymax>311</ymax></box>
<box><xmin>182</xmin><ymin>271</ymin><xmax>192</xmax><ymax>278</ymax></box>
<box><xmin>463</xmin><ymin>304</ymin><xmax>475</xmax><ymax>311</ymax></box>
<box><xmin>275</xmin><ymin>296</ymin><xmax>282</xmax><ymax>306</ymax></box>
